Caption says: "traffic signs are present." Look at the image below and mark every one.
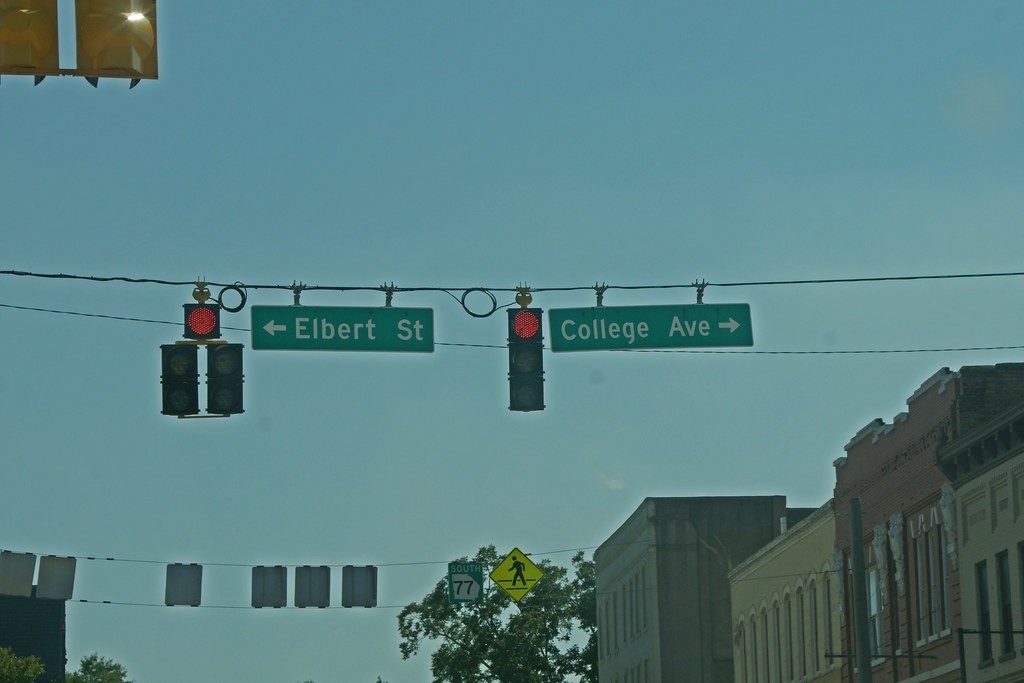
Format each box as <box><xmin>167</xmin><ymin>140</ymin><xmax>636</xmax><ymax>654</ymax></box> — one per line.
<box><xmin>250</xmin><ymin>304</ymin><xmax>435</xmax><ymax>354</ymax></box>
<box><xmin>549</xmin><ymin>303</ymin><xmax>755</xmax><ymax>356</ymax></box>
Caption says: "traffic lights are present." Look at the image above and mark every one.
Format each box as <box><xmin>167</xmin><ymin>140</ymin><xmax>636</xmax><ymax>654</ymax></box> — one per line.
<box><xmin>181</xmin><ymin>303</ymin><xmax>224</xmax><ymax>339</ymax></box>
<box><xmin>205</xmin><ymin>341</ymin><xmax>244</xmax><ymax>415</ymax></box>
<box><xmin>506</xmin><ymin>307</ymin><xmax>547</xmax><ymax>412</ymax></box>
<box><xmin>158</xmin><ymin>343</ymin><xmax>202</xmax><ymax>416</ymax></box>
